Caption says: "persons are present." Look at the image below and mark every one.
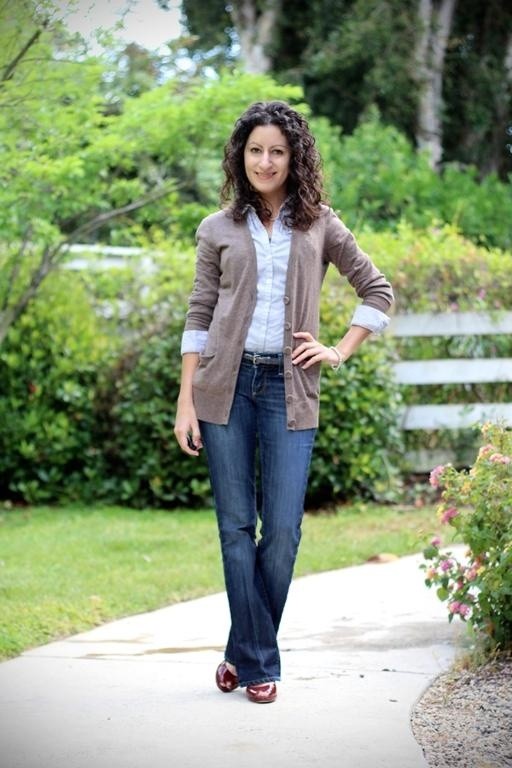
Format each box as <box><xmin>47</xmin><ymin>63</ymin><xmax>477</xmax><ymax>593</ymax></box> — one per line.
<box><xmin>172</xmin><ymin>98</ymin><xmax>397</xmax><ymax>703</ymax></box>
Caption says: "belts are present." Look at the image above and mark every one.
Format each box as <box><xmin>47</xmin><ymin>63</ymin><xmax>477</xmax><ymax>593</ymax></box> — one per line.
<box><xmin>242</xmin><ymin>353</ymin><xmax>283</xmax><ymax>365</ymax></box>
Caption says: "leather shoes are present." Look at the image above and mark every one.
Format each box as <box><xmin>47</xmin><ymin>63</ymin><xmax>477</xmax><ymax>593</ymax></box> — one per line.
<box><xmin>247</xmin><ymin>683</ymin><xmax>277</xmax><ymax>703</ymax></box>
<box><xmin>216</xmin><ymin>660</ymin><xmax>240</xmax><ymax>692</ymax></box>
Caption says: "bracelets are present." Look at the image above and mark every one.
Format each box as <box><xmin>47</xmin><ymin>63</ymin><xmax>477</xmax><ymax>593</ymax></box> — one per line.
<box><xmin>330</xmin><ymin>344</ymin><xmax>342</xmax><ymax>372</ymax></box>
